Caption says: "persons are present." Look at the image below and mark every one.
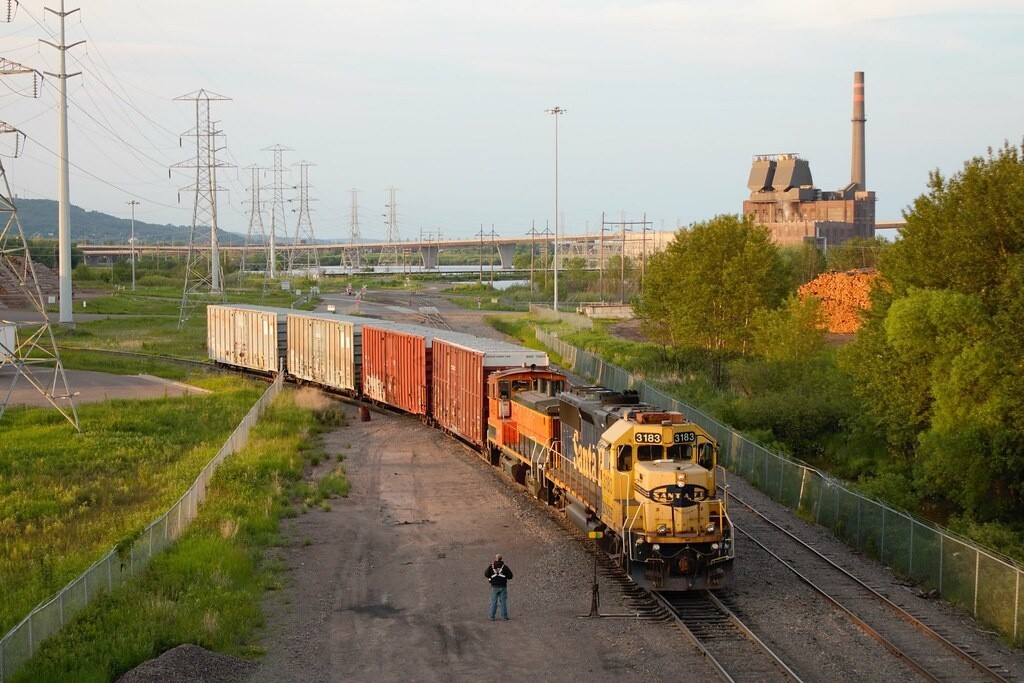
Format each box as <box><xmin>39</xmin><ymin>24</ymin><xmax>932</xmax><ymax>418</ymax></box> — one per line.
<box><xmin>484</xmin><ymin>554</ymin><xmax>513</xmax><ymax>620</ymax></box>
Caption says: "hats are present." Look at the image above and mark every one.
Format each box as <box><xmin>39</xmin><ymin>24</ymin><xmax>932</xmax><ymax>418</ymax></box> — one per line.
<box><xmin>496</xmin><ymin>554</ymin><xmax>502</xmax><ymax>559</ymax></box>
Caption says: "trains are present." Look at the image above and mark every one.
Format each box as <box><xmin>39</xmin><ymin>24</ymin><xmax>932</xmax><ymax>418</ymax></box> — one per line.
<box><xmin>207</xmin><ymin>303</ymin><xmax>737</xmax><ymax>596</ymax></box>
